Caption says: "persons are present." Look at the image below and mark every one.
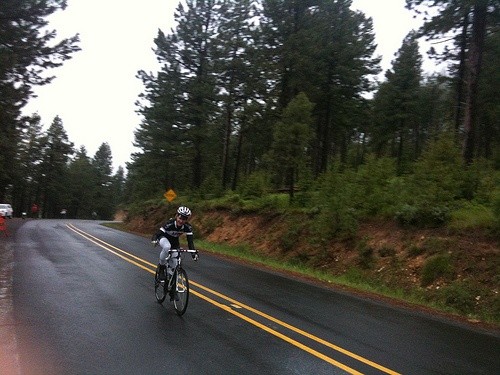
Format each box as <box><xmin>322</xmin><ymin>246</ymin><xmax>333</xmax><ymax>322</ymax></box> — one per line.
<box><xmin>32</xmin><ymin>203</ymin><xmax>39</xmax><ymax>219</ymax></box>
<box><xmin>152</xmin><ymin>206</ymin><xmax>199</xmax><ymax>299</ymax></box>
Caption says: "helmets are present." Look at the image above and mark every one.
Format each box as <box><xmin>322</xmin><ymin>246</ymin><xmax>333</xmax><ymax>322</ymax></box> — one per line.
<box><xmin>177</xmin><ymin>206</ymin><xmax>192</xmax><ymax>219</ymax></box>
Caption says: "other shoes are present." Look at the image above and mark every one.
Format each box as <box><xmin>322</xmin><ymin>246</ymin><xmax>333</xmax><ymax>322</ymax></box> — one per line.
<box><xmin>169</xmin><ymin>293</ymin><xmax>180</xmax><ymax>302</ymax></box>
<box><xmin>156</xmin><ymin>263</ymin><xmax>166</xmax><ymax>280</ymax></box>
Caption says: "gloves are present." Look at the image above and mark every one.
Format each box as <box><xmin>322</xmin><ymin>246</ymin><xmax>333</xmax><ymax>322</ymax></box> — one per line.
<box><xmin>192</xmin><ymin>253</ymin><xmax>200</xmax><ymax>262</ymax></box>
<box><xmin>151</xmin><ymin>239</ymin><xmax>160</xmax><ymax>247</ymax></box>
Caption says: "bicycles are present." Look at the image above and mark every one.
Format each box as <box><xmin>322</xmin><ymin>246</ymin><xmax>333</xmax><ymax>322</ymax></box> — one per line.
<box><xmin>154</xmin><ymin>247</ymin><xmax>198</xmax><ymax>316</ymax></box>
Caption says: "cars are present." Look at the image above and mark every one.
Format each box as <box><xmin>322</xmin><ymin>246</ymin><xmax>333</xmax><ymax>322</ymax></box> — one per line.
<box><xmin>0</xmin><ymin>203</ymin><xmax>14</xmax><ymax>219</ymax></box>
